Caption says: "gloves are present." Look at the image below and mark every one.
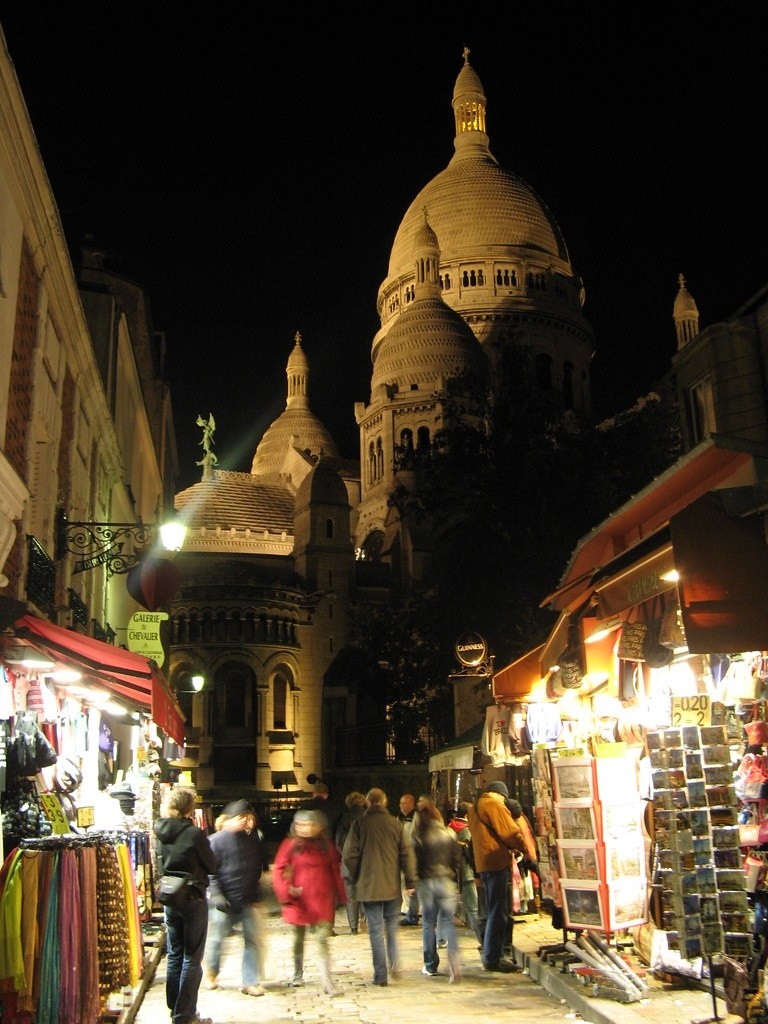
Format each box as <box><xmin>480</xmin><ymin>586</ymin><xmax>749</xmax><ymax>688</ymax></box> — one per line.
<box><xmin>215</xmin><ymin>896</ymin><xmax>232</xmax><ymax>915</ymax></box>
<box><xmin>516</xmin><ymin>853</ymin><xmax>539</xmax><ymax>879</ymax></box>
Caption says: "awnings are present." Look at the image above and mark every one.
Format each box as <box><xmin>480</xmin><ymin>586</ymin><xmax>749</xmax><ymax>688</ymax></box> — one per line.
<box><xmin>596</xmin><ymin>540</ymin><xmax>678</xmax><ymax>622</ymax></box>
<box><xmin>536</xmin><ymin>578</ymin><xmax>600</xmax><ymax>680</ymax></box>
<box><xmin>14</xmin><ymin>615</ymin><xmax>188</xmax><ymax>749</ymax></box>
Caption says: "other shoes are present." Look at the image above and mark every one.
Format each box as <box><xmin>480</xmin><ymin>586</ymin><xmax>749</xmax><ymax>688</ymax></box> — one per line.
<box><xmin>448</xmin><ymin>966</ymin><xmax>460</xmax><ymax>985</ymax></box>
<box><xmin>437</xmin><ymin>939</ymin><xmax>448</xmax><ymax>949</ymax></box>
<box><xmin>293</xmin><ymin>971</ymin><xmax>303</xmax><ymax>986</ymax></box>
<box><xmin>324</xmin><ymin>984</ymin><xmax>344</xmax><ymax>998</ymax></box>
<box><xmin>371</xmin><ymin>980</ymin><xmax>387</xmax><ymax>986</ymax></box>
<box><xmin>482</xmin><ymin>959</ymin><xmax>519</xmax><ymax>971</ymax></box>
<box><xmin>352</xmin><ymin>928</ymin><xmax>358</xmax><ymax>935</ymax></box>
<box><xmin>400</xmin><ymin>918</ymin><xmax>418</xmax><ymax>926</ymax></box>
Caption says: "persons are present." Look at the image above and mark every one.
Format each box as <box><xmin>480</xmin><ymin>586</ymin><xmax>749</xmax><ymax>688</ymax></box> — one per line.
<box><xmin>341</xmin><ymin>787</ymin><xmax>463</xmax><ymax>987</ymax></box>
<box><xmin>153</xmin><ymin>791</ymin><xmax>218</xmax><ymax>1024</ymax></box>
<box><xmin>207</xmin><ymin>799</ymin><xmax>345</xmax><ymax>997</ymax></box>
<box><xmin>397</xmin><ymin>794</ymin><xmax>481</xmax><ymax>949</ymax></box>
<box><xmin>467</xmin><ymin>782</ymin><xmax>547</xmax><ymax>973</ymax></box>
<box><xmin>291</xmin><ymin>783</ymin><xmax>368</xmax><ymax>936</ymax></box>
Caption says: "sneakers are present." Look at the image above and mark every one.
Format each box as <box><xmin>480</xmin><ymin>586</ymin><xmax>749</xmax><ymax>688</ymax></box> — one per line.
<box><xmin>242</xmin><ymin>985</ymin><xmax>264</xmax><ymax>996</ymax></box>
<box><xmin>172</xmin><ymin>1011</ymin><xmax>213</xmax><ymax>1024</ymax></box>
<box><xmin>421</xmin><ymin>966</ymin><xmax>439</xmax><ymax>975</ymax></box>
<box><xmin>205</xmin><ymin>970</ymin><xmax>217</xmax><ymax>990</ymax></box>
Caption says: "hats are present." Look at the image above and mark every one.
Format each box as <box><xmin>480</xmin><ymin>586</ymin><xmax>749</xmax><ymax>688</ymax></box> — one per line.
<box><xmin>225</xmin><ymin>799</ymin><xmax>255</xmax><ymax>820</ymax></box>
<box><xmin>487</xmin><ymin>781</ymin><xmax>508</xmax><ymax>798</ymax></box>
<box><xmin>505</xmin><ymin>799</ymin><xmax>522</xmax><ymax>819</ymax></box>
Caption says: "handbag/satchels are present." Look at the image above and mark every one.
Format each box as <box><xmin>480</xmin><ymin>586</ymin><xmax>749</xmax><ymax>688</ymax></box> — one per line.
<box><xmin>155</xmin><ymin>876</ymin><xmax>194</xmax><ymax>908</ymax></box>
<box><xmin>16</xmin><ymin>722</ymin><xmax>58</xmax><ymax>777</ymax></box>
<box><xmin>546</xmin><ymin>624</ymin><xmax>584</xmax><ymax>701</ymax></box>
<box><xmin>617</xmin><ymin>588</ymin><xmax>686</xmax><ymax>668</ymax></box>
